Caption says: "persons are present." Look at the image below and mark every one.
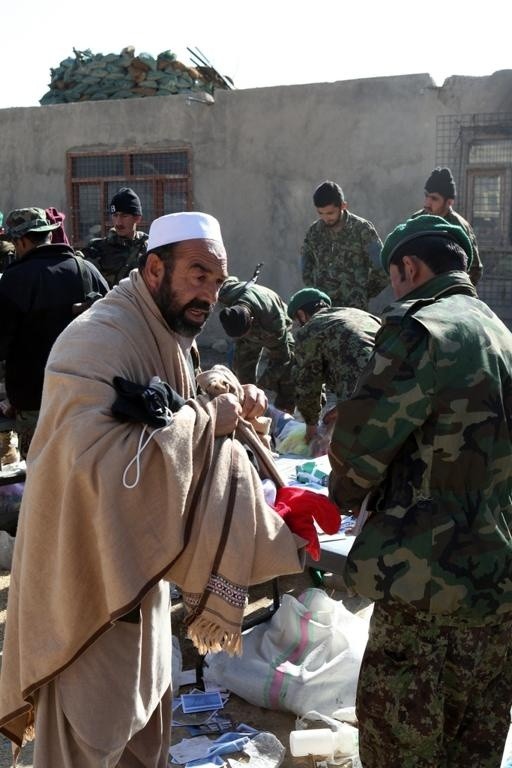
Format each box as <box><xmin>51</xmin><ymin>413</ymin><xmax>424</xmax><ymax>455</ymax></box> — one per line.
<box><xmin>0</xmin><ymin>212</ymin><xmax>39</xmax><ymax>469</ymax></box>
<box><xmin>398</xmin><ymin>168</ymin><xmax>483</xmax><ymax>306</ymax></box>
<box><xmin>327</xmin><ymin>213</ymin><xmax>512</xmax><ymax>767</ymax></box>
<box><xmin>215</xmin><ymin>269</ymin><xmax>302</xmax><ymax>418</ymax></box>
<box><xmin>284</xmin><ymin>289</ymin><xmax>388</xmax><ymax>446</ymax></box>
<box><xmin>0</xmin><ymin>207</ymin><xmax>115</xmax><ymax>473</ymax></box>
<box><xmin>0</xmin><ymin>213</ymin><xmax>267</xmax><ymax>768</ymax></box>
<box><xmin>78</xmin><ymin>188</ymin><xmax>157</xmax><ymax>296</ymax></box>
<box><xmin>296</xmin><ymin>181</ymin><xmax>392</xmax><ymax>309</ymax></box>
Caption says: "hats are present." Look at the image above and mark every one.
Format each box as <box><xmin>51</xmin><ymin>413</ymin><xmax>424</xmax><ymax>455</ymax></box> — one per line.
<box><xmin>381</xmin><ymin>214</ymin><xmax>472</xmax><ymax>275</ymax></box>
<box><xmin>220</xmin><ymin>307</ymin><xmax>250</xmax><ymax>335</ymax></box>
<box><xmin>287</xmin><ymin>288</ymin><xmax>332</xmax><ymax>319</ymax></box>
<box><xmin>425</xmin><ymin>168</ymin><xmax>457</xmax><ymax>199</ymax></box>
<box><xmin>6</xmin><ymin>208</ymin><xmax>59</xmax><ymax>239</ymax></box>
<box><xmin>110</xmin><ymin>188</ymin><xmax>142</xmax><ymax>215</ymax></box>
<box><xmin>146</xmin><ymin>212</ymin><xmax>224</xmax><ymax>250</ymax></box>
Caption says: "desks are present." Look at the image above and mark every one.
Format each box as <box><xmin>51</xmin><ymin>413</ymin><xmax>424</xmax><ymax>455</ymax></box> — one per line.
<box><xmin>196</xmin><ymin>455</ymin><xmax>356</xmax><ymax>692</ymax></box>
